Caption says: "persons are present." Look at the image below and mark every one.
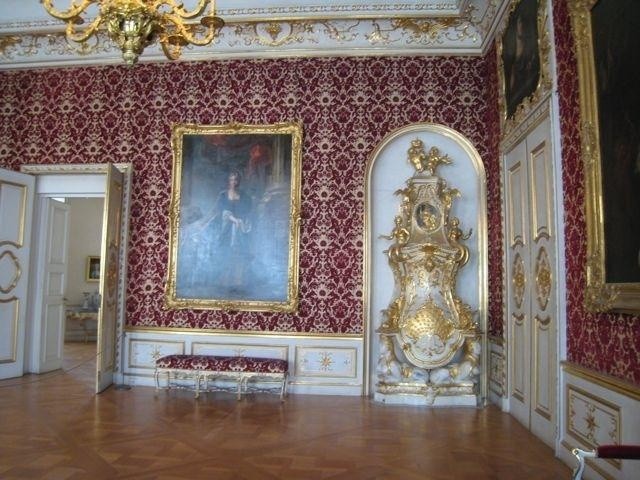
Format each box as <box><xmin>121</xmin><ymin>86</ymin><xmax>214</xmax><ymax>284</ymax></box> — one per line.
<box><xmin>210</xmin><ymin>168</ymin><xmax>257</xmax><ymax>295</ymax></box>
<box><xmin>91</xmin><ymin>262</ymin><xmax>99</xmax><ymax>278</ymax></box>
<box><xmin>447</xmin><ymin>216</ymin><xmax>473</xmax><ymax>267</ymax></box>
<box><xmin>378</xmin><ymin>215</ymin><xmax>411</xmax><ymax>263</ymax></box>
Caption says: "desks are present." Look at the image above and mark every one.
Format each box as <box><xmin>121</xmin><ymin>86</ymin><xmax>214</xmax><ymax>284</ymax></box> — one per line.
<box><xmin>65</xmin><ymin>311</ymin><xmax>98</xmax><ymax>344</ymax></box>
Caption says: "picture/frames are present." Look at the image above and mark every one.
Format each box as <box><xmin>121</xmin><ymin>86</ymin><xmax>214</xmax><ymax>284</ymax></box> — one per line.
<box><xmin>86</xmin><ymin>256</ymin><xmax>100</xmax><ymax>282</ymax></box>
<box><xmin>163</xmin><ymin>120</ymin><xmax>304</xmax><ymax>314</ymax></box>
<box><xmin>564</xmin><ymin>1</ymin><xmax>639</xmax><ymax>315</ymax></box>
<box><xmin>493</xmin><ymin>0</ymin><xmax>553</xmax><ymax>140</ymax></box>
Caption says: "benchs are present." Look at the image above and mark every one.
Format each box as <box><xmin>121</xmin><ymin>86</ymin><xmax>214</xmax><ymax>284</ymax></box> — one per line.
<box><xmin>155</xmin><ymin>354</ymin><xmax>287</xmax><ymax>402</ymax></box>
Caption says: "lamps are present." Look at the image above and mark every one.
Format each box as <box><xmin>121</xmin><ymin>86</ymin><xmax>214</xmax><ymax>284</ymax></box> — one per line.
<box><xmin>41</xmin><ymin>1</ymin><xmax>224</xmax><ymax>68</ymax></box>
